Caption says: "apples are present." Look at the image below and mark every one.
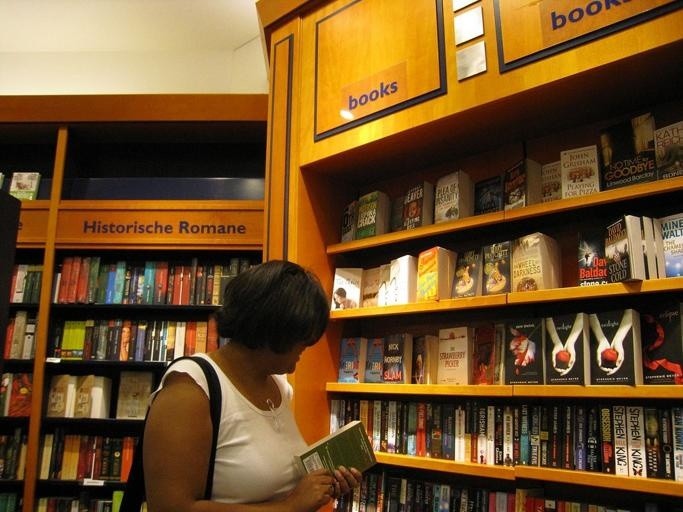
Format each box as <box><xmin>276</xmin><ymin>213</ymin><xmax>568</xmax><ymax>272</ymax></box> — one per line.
<box><xmin>601</xmin><ymin>349</ymin><xmax>619</xmax><ymax>367</ymax></box>
<box><xmin>556</xmin><ymin>351</ymin><xmax>571</xmax><ymax>368</ymax></box>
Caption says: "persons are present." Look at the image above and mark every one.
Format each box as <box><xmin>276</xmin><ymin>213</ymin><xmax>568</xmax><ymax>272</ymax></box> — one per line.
<box><xmin>141</xmin><ymin>259</ymin><xmax>362</xmax><ymax>511</ymax></box>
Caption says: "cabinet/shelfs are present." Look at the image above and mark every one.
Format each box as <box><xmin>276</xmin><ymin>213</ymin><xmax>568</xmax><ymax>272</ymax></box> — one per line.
<box><xmin>256</xmin><ymin>0</ymin><xmax>683</xmax><ymax>512</ymax></box>
<box><xmin>1</xmin><ymin>93</ymin><xmax>269</xmax><ymax>510</ymax></box>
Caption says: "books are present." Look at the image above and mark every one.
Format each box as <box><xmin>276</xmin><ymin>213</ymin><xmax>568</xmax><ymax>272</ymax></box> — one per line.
<box><xmin>0</xmin><ymin>172</ymin><xmax>5</xmax><ymax>189</ymax></box>
<box><xmin>9</xmin><ymin>171</ymin><xmax>42</xmax><ymax>201</ymax></box>
<box><xmin>293</xmin><ymin>420</ymin><xmax>377</xmax><ymax>483</ymax></box>
